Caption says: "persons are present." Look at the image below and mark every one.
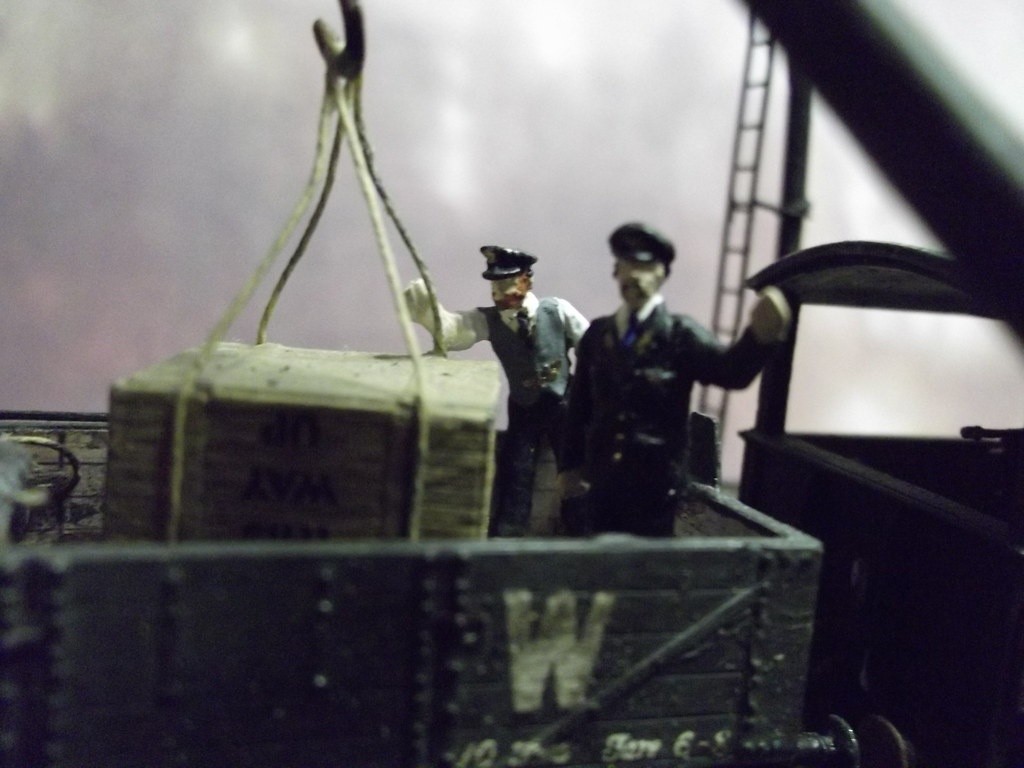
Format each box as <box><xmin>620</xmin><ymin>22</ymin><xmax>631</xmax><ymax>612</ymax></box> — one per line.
<box><xmin>550</xmin><ymin>224</ymin><xmax>791</xmax><ymax>537</ymax></box>
<box><xmin>404</xmin><ymin>243</ymin><xmax>600</xmax><ymax>539</ymax></box>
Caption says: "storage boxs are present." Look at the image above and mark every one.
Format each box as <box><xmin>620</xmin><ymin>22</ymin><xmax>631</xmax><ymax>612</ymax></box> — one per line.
<box><xmin>99</xmin><ymin>338</ymin><xmax>499</xmax><ymax>544</ymax></box>
